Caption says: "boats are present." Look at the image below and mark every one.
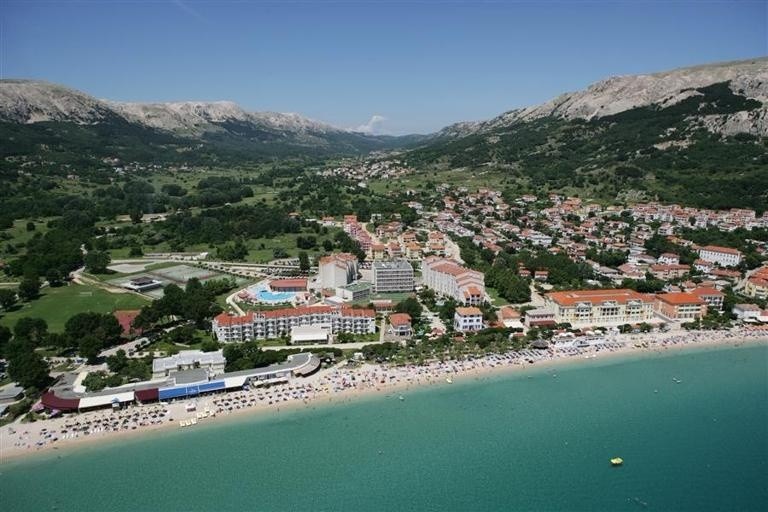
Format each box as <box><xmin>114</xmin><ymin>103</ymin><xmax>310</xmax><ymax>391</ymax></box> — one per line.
<box><xmin>610</xmin><ymin>456</ymin><xmax>623</xmax><ymax>466</ymax></box>
